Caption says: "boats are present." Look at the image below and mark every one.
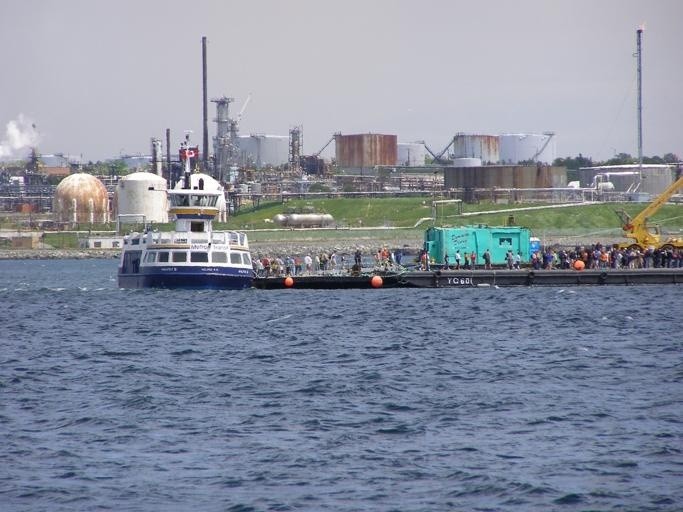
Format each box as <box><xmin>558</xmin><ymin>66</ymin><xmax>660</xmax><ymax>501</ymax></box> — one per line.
<box><xmin>117</xmin><ymin>128</ymin><xmax>255</xmax><ymax>291</ymax></box>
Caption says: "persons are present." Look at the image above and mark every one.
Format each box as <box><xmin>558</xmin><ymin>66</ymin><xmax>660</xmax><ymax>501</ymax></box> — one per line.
<box><xmin>252</xmin><ymin>248</ymin><xmax>338</xmax><ymax>277</ymax></box>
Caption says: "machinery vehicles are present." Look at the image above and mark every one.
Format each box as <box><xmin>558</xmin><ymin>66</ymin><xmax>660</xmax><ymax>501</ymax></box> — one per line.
<box><xmin>616</xmin><ymin>175</ymin><xmax>682</xmax><ymax>256</ymax></box>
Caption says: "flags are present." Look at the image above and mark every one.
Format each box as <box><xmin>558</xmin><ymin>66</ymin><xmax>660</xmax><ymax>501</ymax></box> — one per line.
<box><xmin>179</xmin><ymin>148</ymin><xmax>199</xmax><ymax>160</ymax></box>
